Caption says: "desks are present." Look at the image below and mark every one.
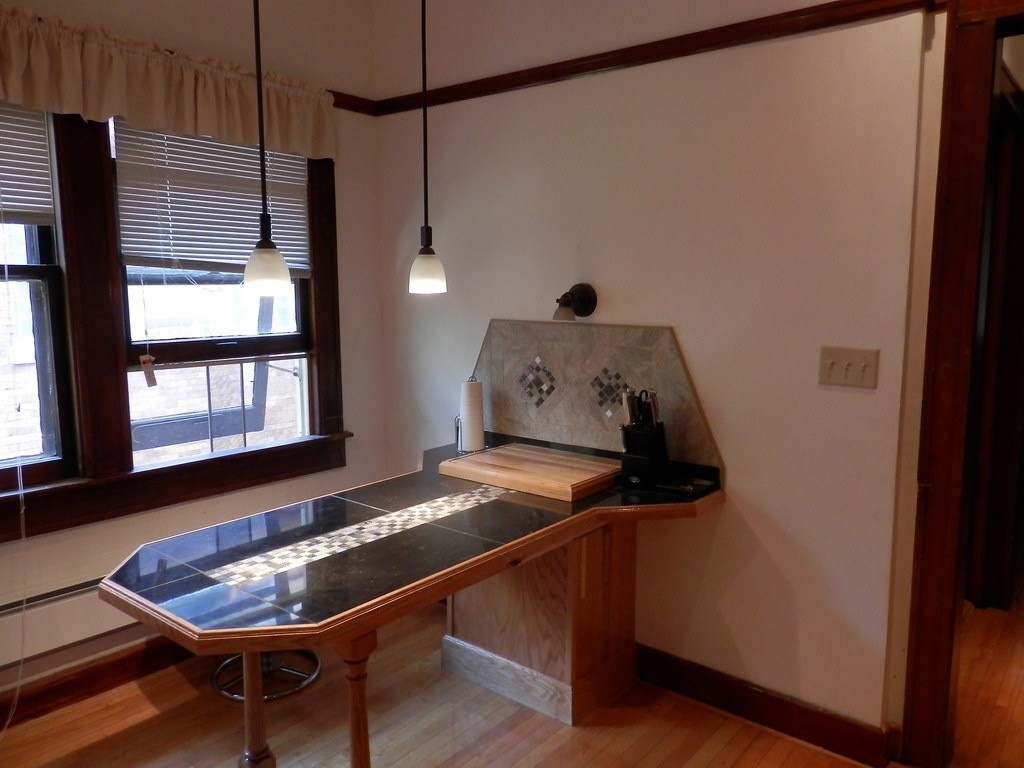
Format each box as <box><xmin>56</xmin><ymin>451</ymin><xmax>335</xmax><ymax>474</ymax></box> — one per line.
<box><xmin>97</xmin><ymin>441</ymin><xmax>726</xmax><ymax>768</ymax></box>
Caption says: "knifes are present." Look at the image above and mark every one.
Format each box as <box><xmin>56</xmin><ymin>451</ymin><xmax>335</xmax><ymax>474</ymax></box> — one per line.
<box><xmin>622</xmin><ymin>385</ymin><xmax>660</xmax><ymax>428</ymax></box>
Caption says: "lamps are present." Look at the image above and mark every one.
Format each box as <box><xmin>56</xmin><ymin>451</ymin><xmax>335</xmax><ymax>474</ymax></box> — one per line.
<box><xmin>408</xmin><ymin>0</ymin><xmax>447</xmax><ymax>293</ymax></box>
<box><xmin>244</xmin><ymin>0</ymin><xmax>293</xmax><ymax>297</ymax></box>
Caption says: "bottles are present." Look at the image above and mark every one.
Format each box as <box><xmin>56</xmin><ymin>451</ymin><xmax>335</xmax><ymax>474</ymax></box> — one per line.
<box><xmin>457</xmin><ymin>375</ymin><xmax>485</xmax><ymax>451</ymax></box>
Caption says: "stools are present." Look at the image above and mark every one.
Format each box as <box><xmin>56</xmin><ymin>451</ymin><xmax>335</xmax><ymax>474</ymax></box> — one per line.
<box><xmin>212</xmin><ymin>648</ymin><xmax>322</xmax><ymax>703</ymax></box>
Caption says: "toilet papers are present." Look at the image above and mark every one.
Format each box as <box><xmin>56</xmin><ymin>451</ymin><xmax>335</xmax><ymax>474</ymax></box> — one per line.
<box><xmin>456</xmin><ymin>380</ymin><xmax>485</xmax><ymax>453</ymax></box>
